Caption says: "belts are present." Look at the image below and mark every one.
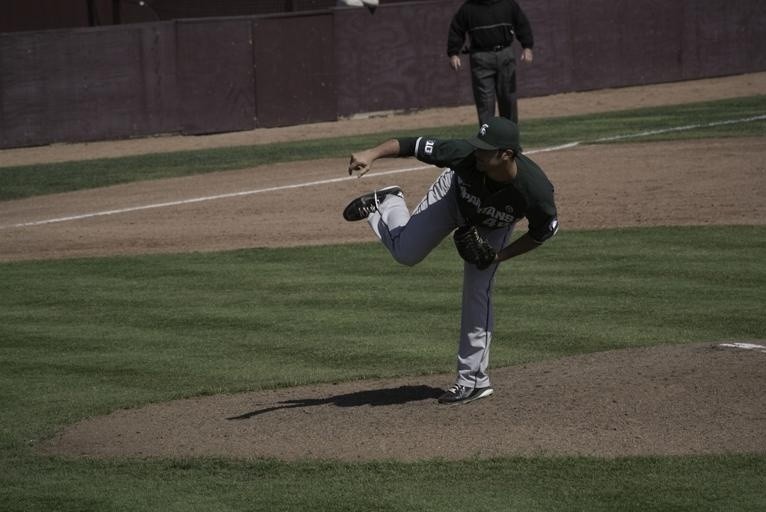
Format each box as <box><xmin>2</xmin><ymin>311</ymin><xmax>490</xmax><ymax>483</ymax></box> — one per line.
<box><xmin>469</xmin><ymin>42</ymin><xmax>511</xmax><ymax>52</ymax></box>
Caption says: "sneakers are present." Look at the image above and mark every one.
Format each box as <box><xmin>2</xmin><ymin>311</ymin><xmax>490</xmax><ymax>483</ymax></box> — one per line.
<box><xmin>437</xmin><ymin>381</ymin><xmax>493</xmax><ymax>406</ymax></box>
<box><xmin>342</xmin><ymin>185</ymin><xmax>403</xmax><ymax>222</ymax></box>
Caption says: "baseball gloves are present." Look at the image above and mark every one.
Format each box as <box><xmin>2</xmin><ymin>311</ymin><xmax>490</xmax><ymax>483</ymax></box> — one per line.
<box><xmin>453</xmin><ymin>222</ymin><xmax>495</xmax><ymax>271</ymax></box>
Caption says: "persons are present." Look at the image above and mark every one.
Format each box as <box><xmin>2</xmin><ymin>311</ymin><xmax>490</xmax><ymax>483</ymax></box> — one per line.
<box><xmin>446</xmin><ymin>0</ymin><xmax>534</xmax><ymax>126</ymax></box>
<box><xmin>342</xmin><ymin>117</ymin><xmax>558</xmax><ymax>408</ymax></box>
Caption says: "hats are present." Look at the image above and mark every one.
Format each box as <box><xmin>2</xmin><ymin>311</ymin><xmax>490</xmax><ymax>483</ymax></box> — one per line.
<box><xmin>463</xmin><ymin>116</ymin><xmax>519</xmax><ymax>152</ymax></box>
